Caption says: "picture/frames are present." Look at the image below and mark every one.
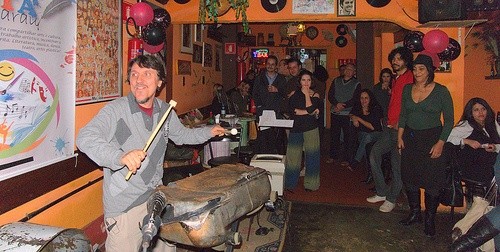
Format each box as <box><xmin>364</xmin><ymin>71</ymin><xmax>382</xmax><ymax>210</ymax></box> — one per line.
<box><xmin>214</xmin><ymin>44</ymin><xmax>221</xmax><ymax>72</ymax></box>
<box><xmin>337</xmin><ymin>0</ymin><xmax>356</xmax><ymax>17</ymax></box>
<box><xmin>179</xmin><ymin>24</ymin><xmax>194</xmax><ymax>54</ymax></box>
<box><xmin>224</xmin><ymin>41</ymin><xmax>237</xmax><ymax>55</ymax></box>
<box><xmin>434</xmin><ymin>61</ymin><xmax>451</xmax><ymax>73</ymax></box>
<box><xmin>75</xmin><ymin>0</ymin><xmax>124</xmax><ymax>106</ymax></box>
<box><xmin>193</xmin><ymin>23</ymin><xmax>204</xmax><ymax>46</ymax></box>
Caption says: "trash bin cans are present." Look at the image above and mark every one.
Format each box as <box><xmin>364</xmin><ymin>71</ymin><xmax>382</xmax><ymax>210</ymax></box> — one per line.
<box><xmin>201</xmin><ymin>138</ymin><xmax>232</xmax><ymax>169</ymax></box>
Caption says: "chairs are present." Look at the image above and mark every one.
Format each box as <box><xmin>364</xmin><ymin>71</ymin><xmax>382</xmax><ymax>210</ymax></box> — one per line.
<box><xmin>162</xmin><ymin>144</ymin><xmax>204</xmax><ymax>187</ymax></box>
<box><xmin>449</xmin><ymin>143</ymin><xmax>490</xmax><ymax>217</ymax></box>
<box><xmin>208</xmin><ymin>126</ymin><xmax>243</xmax><ymax>169</ymax></box>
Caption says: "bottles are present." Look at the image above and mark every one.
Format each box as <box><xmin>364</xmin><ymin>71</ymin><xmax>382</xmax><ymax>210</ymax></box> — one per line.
<box><xmin>220</xmin><ymin>104</ymin><xmax>226</xmax><ymax>119</ymax></box>
<box><xmin>257</xmin><ymin>32</ymin><xmax>264</xmax><ymax>46</ymax></box>
<box><xmin>207</xmin><ymin>112</ymin><xmax>215</xmax><ymax>125</ymax></box>
<box><xmin>267</xmin><ymin>32</ymin><xmax>274</xmax><ymax>46</ymax></box>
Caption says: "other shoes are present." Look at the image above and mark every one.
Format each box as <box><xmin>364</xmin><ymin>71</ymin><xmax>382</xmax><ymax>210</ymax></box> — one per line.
<box><xmin>366</xmin><ymin>195</ymin><xmax>387</xmax><ymax>203</ymax></box>
<box><xmin>379</xmin><ymin>199</ymin><xmax>396</xmax><ymax>213</ymax></box>
<box><xmin>345</xmin><ymin>167</ymin><xmax>353</xmax><ymax>175</ymax></box>
<box><xmin>325</xmin><ymin>157</ymin><xmax>336</xmax><ymax>164</ymax></box>
<box><xmin>341</xmin><ymin>160</ymin><xmax>351</xmax><ymax>167</ymax></box>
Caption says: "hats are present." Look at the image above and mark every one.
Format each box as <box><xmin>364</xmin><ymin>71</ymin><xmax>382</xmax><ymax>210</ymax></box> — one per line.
<box><xmin>410</xmin><ymin>54</ymin><xmax>437</xmax><ymax>72</ymax></box>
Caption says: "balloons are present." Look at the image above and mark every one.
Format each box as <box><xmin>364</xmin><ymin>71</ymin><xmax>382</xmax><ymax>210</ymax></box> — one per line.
<box><xmin>403</xmin><ymin>29</ymin><xmax>461</xmax><ymax>69</ymax></box>
<box><xmin>155</xmin><ymin>0</ymin><xmax>191</xmax><ymax>5</ymax></box>
<box><xmin>130</xmin><ymin>2</ymin><xmax>171</xmax><ymax>53</ymax></box>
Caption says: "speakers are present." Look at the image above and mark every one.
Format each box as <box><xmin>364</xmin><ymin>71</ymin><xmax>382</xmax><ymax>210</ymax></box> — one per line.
<box><xmin>418</xmin><ymin>0</ymin><xmax>462</xmax><ymax>23</ymax></box>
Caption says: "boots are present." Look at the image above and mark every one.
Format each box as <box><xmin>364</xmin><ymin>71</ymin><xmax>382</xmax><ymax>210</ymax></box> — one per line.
<box><xmin>398</xmin><ymin>189</ymin><xmax>424</xmax><ymax>226</ymax></box>
<box><xmin>424</xmin><ymin>191</ymin><xmax>441</xmax><ymax>239</ymax></box>
<box><xmin>448</xmin><ymin>195</ymin><xmax>495</xmax><ymax>252</ymax></box>
<box><xmin>360</xmin><ymin>161</ymin><xmax>374</xmax><ymax>184</ymax></box>
<box><xmin>368</xmin><ymin>166</ymin><xmax>392</xmax><ymax>192</ymax></box>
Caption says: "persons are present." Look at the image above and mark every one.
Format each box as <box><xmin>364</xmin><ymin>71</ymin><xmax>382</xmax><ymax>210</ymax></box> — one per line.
<box><xmin>339</xmin><ymin>65</ymin><xmax>347</xmax><ymax>77</ymax></box>
<box><xmin>397</xmin><ymin>54</ymin><xmax>454</xmax><ymax>237</ymax></box>
<box><xmin>348</xmin><ymin>89</ymin><xmax>384</xmax><ymax>183</ymax></box>
<box><xmin>447</xmin><ymin>153</ymin><xmax>500</xmax><ymax>252</ymax></box>
<box><xmin>252</xmin><ymin>55</ymin><xmax>288</xmax><ymax>119</ymax></box>
<box><xmin>267</xmin><ymin>57</ymin><xmax>325</xmax><ymax>170</ymax></box>
<box><xmin>243</xmin><ymin>70</ymin><xmax>256</xmax><ymax>88</ymax></box>
<box><xmin>285</xmin><ymin>69</ymin><xmax>322</xmax><ymax>191</ymax></box>
<box><xmin>445</xmin><ymin>97</ymin><xmax>500</xmax><ymax>186</ymax></box>
<box><xmin>366</xmin><ymin>47</ymin><xmax>415</xmax><ymax>212</ymax></box>
<box><xmin>371</xmin><ymin>68</ymin><xmax>392</xmax><ymax>115</ymax></box>
<box><xmin>230</xmin><ymin>78</ymin><xmax>253</xmax><ymax>117</ymax></box>
<box><xmin>325</xmin><ymin>63</ymin><xmax>362</xmax><ymax>166</ymax></box>
<box><xmin>278</xmin><ymin>58</ymin><xmax>295</xmax><ymax>83</ymax></box>
<box><xmin>76</xmin><ymin>54</ymin><xmax>228</xmax><ymax>252</ymax></box>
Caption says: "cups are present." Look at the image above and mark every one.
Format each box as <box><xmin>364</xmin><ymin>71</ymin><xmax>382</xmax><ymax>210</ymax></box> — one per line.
<box><xmin>230</xmin><ymin>116</ymin><xmax>235</xmax><ymax>128</ymax></box>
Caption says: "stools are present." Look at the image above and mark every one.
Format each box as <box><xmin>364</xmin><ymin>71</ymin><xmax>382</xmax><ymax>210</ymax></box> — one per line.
<box><xmin>234</xmin><ymin>146</ymin><xmax>254</xmax><ymax>165</ymax></box>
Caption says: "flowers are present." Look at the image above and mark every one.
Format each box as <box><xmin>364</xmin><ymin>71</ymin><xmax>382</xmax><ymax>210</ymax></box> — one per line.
<box><xmin>470</xmin><ymin>10</ymin><xmax>500</xmax><ymax>56</ymax></box>
<box><xmin>485</xmin><ymin>56</ymin><xmax>496</xmax><ymax>64</ymax></box>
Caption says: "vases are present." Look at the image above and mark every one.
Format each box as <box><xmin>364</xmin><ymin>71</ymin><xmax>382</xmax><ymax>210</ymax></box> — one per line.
<box><xmin>491</xmin><ymin>64</ymin><xmax>494</xmax><ymax>76</ymax></box>
<box><xmin>257</xmin><ymin>33</ymin><xmax>264</xmax><ymax>46</ymax></box>
<box><xmin>495</xmin><ymin>57</ymin><xmax>500</xmax><ymax>76</ymax></box>
